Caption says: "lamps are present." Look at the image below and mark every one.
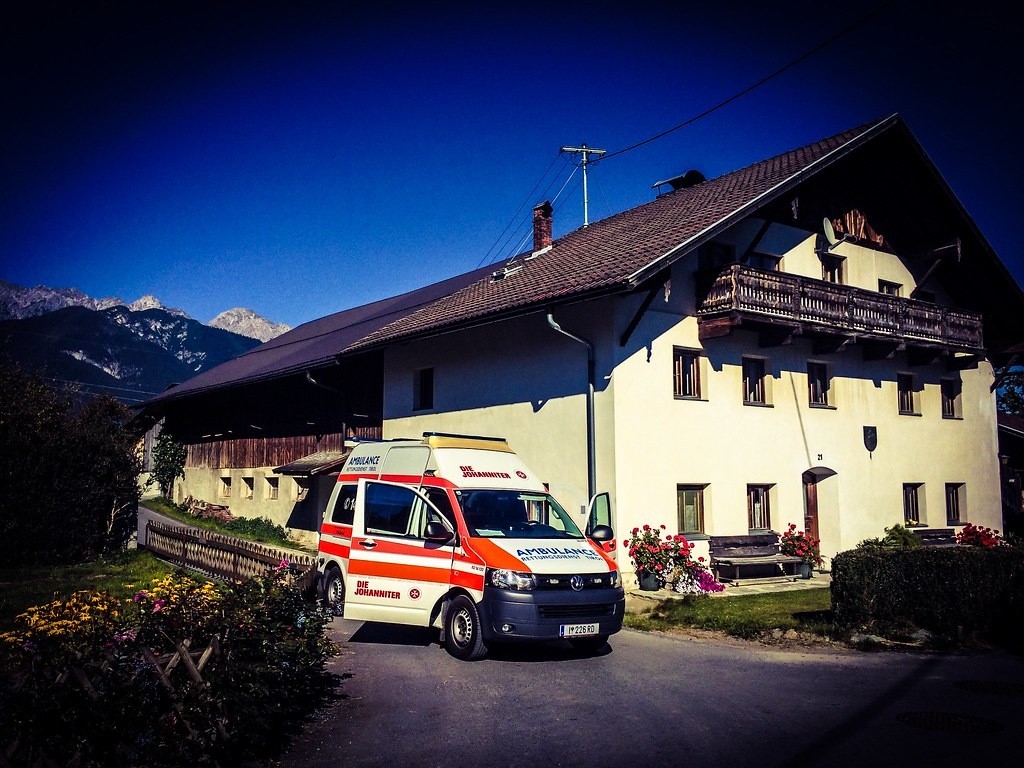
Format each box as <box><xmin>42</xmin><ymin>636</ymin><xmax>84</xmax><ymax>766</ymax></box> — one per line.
<box><xmin>998</xmin><ymin>453</ymin><xmax>1009</xmax><ymax>465</ymax></box>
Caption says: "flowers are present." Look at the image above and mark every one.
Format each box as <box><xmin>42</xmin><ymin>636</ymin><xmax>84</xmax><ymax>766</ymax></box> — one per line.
<box><xmin>671</xmin><ymin>557</ymin><xmax>725</xmax><ymax>595</ymax></box>
<box><xmin>778</xmin><ymin>523</ymin><xmax>828</xmax><ymax>571</ymax></box>
<box><xmin>623</xmin><ymin>524</ymin><xmax>695</xmax><ymax>574</ymax></box>
<box><xmin>956</xmin><ymin>525</ymin><xmax>1001</xmax><ymax>549</ymax></box>
<box><xmin>905</xmin><ymin>519</ymin><xmax>918</xmax><ymax>527</ymax></box>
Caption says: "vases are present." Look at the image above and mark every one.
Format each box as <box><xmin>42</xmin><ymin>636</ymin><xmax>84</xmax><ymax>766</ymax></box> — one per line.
<box><xmin>638</xmin><ymin>569</ymin><xmax>660</xmax><ymax>591</ymax></box>
<box><xmin>797</xmin><ymin>564</ymin><xmax>809</xmax><ymax>579</ymax></box>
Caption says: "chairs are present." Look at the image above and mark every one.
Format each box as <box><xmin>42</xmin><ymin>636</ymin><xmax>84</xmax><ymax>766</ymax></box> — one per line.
<box><xmin>494</xmin><ymin>495</ymin><xmax>521</xmax><ymax>530</ymax></box>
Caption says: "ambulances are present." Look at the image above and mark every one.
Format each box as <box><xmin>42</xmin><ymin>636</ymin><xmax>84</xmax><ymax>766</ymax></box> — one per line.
<box><xmin>315</xmin><ymin>431</ymin><xmax>626</xmax><ymax>662</ymax></box>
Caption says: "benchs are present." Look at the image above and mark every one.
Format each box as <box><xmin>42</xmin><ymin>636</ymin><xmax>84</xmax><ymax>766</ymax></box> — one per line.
<box><xmin>912</xmin><ymin>529</ymin><xmax>957</xmax><ymax>545</ymax></box>
<box><xmin>708</xmin><ymin>534</ymin><xmax>803</xmax><ymax>587</ymax></box>
